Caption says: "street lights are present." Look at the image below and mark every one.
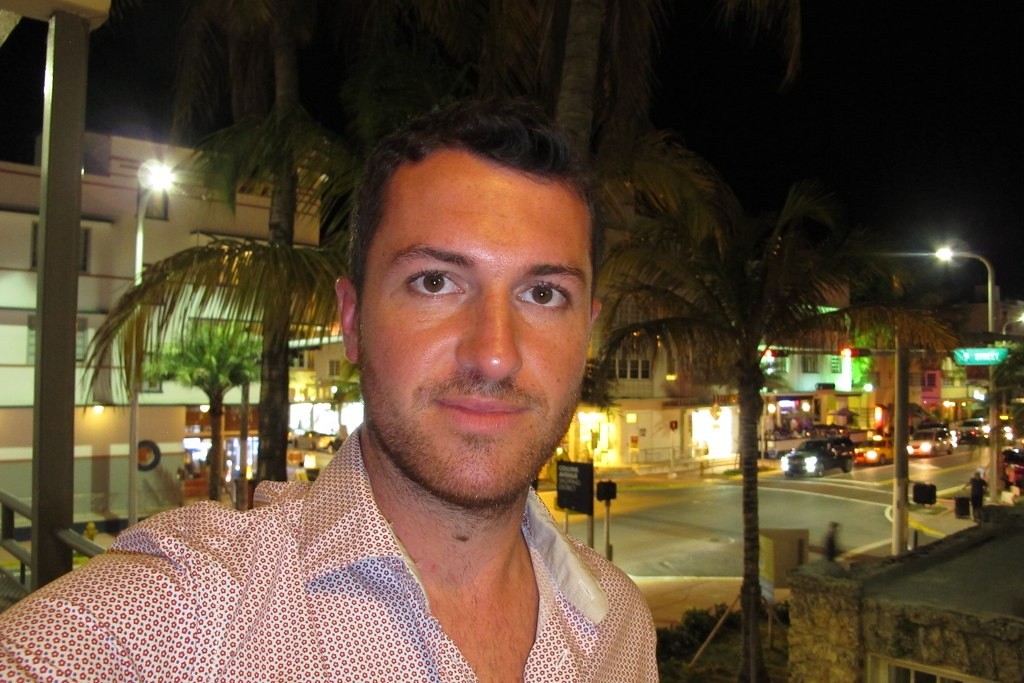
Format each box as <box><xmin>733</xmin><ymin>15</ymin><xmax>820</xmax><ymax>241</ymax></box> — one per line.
<box><xmin>933</xmin><ymin>247</ymin><xmax>1004</xmax><ymax>504</ymax></box>
<box><xmin>124</xmin><ymin>171</ymin><xmax>176</xmax><ymax>528</ymax></box>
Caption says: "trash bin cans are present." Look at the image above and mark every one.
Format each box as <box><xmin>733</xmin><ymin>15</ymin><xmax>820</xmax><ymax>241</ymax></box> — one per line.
<box><xmin>954</xmin><ymin>496</ymin><xmax>970</xmax><ymax>517</ymax></box>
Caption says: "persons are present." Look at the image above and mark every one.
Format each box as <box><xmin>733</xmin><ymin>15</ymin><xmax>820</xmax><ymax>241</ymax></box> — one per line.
<box><xmin>962</xmin><ymin>471</ymin><xmax>989</xmax><ymax>522</ymax></box>
<box><xmin>0</xmin><ymin>102</ymin><xmax>661</xmax><ymax>683</ymax></box>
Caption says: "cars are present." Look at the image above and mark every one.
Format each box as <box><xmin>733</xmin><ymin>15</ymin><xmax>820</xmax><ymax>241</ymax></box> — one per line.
<box><xmin>906</xmin><ymin>426</ymin><xmax>954</xmax><ymax>458</ymax></box>
<box><xmin>855</xmin><ymin>436</ymin><xmax>895</xmax><ymax>466</ymax></box>
<box><xmin>955</xmin><ymin>416</ymin><xmax>990</xmax><ymax>445</ymax></box>
<box><xmin>983</xmin><ymin>447</ymin><xmax>1024</xmax><ymax>494</ymax></box>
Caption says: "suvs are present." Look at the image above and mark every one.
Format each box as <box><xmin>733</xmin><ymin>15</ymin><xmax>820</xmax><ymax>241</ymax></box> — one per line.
<box><xmin>782</xmin><ymin>434</ymin><xmax>855</xmax><ymax>478</ymax></box>
